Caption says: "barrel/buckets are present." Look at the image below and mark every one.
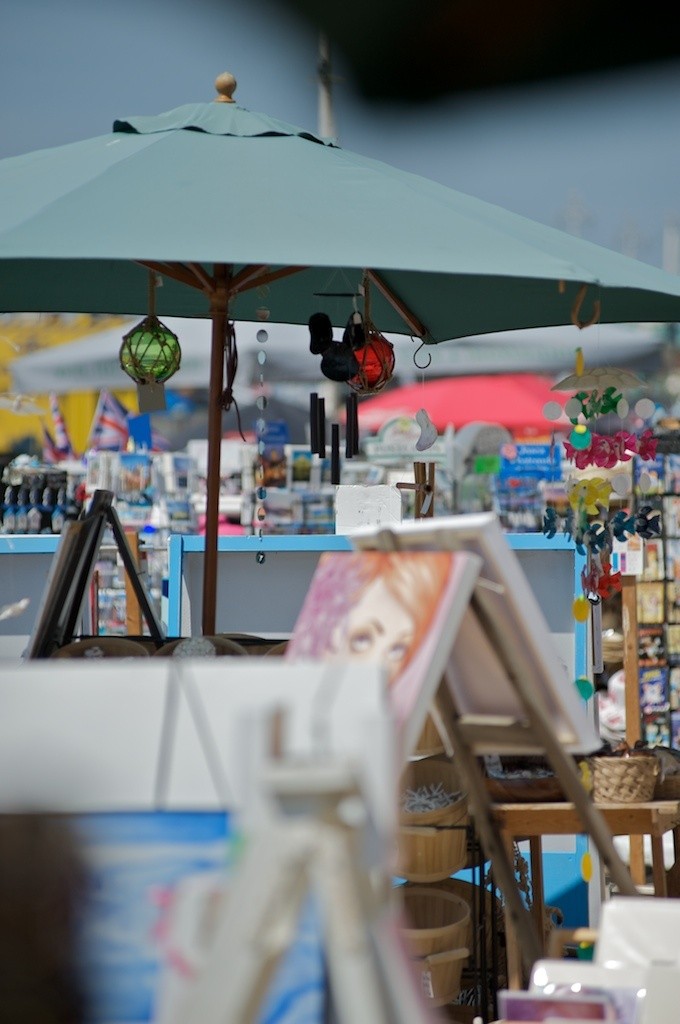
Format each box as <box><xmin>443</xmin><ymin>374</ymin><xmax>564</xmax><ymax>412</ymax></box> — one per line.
<box><xmin>372</xmin><ymin>674</ymin><xmax>508</xmax><ymax>1024</ymax></box>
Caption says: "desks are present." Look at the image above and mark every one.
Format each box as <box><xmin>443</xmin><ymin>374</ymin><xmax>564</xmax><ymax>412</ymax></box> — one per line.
<box><xmin>487</xmin><ymin>798</ymin><xmax>680</xmax><ymax>992</ymax></box>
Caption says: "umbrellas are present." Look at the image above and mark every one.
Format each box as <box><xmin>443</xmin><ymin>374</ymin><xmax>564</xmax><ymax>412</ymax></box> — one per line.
<box><xmin>0</xmin><ymin>70</ymin><xmax>680</xmax><ymax>638</ymax></box>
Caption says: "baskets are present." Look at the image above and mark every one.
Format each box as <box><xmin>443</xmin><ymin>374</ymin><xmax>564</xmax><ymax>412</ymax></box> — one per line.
<box><xmin>590</xmin><ymin>755</ymin><xmax>658</xmax><ymax>803</ymax></box>
<box><xmin>656</xmin><ymin>773</ymin><xmax>680</xmax><ymax>800</ymax></box>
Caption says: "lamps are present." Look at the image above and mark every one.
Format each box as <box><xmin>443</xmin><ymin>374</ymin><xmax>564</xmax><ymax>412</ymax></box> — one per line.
<box><xmin>119</xmin><ymin>269</ymin><xmax>181</xmax><ymax>386</ymax></box>
<box><xmin>339</xmin><ymin>295</ymin><xmax>396</xmax><ymax>395</ymax></box>
